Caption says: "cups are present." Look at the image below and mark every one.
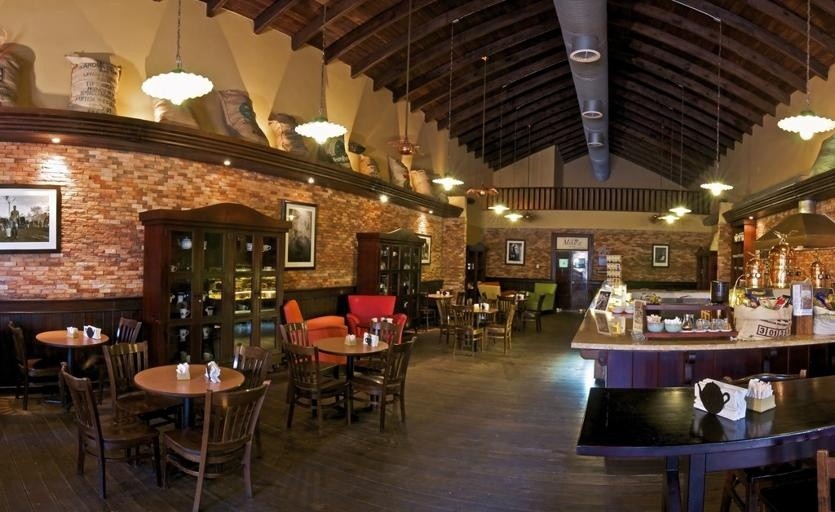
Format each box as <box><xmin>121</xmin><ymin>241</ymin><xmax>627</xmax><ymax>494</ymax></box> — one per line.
<box><xmin>180</xmin><ymin>330</ymin><xmax>189</xmax><ymax>336</ymax></box>
<box><xmin>695</xmin><ymin>318</ymin><xmax>708</xmax><ymax>330</ymax></box>
<box><xmin>246</xmin><ymin>242</ymin><xmax>252</xmax><ymax>251</ymax></box>
<box><xmin>205</xmin><ymin>306</ymin><xmax>214</xmax><ymax>314</ymax></box>
<box><xmin>263</xmin><ymin>244</ymin><xmax>272</xmax><ymax>252</ymax></box>
<box><xmin>203</xmin><ymin>327</ymin><xmax>211</xmax><ymax>336</ymax></box>
<box><xmin>202</xmin><ymin>352</ymin><xmax>213</xmax><ymax>360</ymax></box>
<box><xmin>180</xmin><ymin>309</ymin><xmax>189</xmax><ymax>319</ymax></box>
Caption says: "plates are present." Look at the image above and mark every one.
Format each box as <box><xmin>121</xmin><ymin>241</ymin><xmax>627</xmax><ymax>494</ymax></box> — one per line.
<box><xmin>707</xmin><ymin>329</ymin><xmax>720</xmax><ymax>332</ymax></box>
<box><xmin>721</xmin><ymin>328</ymin><xmax>732</xmax><ymax>332</ymax></box>
<box><xmin>681</xmin><ymin>329</ymin><xmax>694</xmax><ymax>333</ymax></box>
<box><xmin>695</xmin><ymin>329</ymin><xmax>706</xmax><ymax>332</ymax></box>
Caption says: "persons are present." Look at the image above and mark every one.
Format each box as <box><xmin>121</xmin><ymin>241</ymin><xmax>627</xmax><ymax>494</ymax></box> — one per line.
<box><xmin>510</xmin><ymin>243</ymin><xmax>522</xmax><ymax>262</ymax></box>
<box><xmin>466</xmin><ymin>282</ymin><xmax>478</xmax><ymax>302</ymax></box>
<box><xmin>9</xmin><ymin>205</ymin><xmax>21</xmax><ymax>241</ymax></box>
<box><xmin>289</xmin><ymin>215</ymin><xmax>310</xmax><ymax>261</ymax></box>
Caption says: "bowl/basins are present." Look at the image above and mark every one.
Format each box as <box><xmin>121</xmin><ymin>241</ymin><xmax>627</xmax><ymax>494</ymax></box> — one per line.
<box><xmin>665</xmin><ymin>323</ymin><xmax>683</xmax><ymax>333</ymax></box>
<box><xmin>647</xmin><ymin>323</ymin><xmax>664</xmax><ymax>333</ymax></box>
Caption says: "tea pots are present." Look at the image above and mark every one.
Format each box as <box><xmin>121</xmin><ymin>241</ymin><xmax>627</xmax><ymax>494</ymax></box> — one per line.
<box><xmin>176</xmin><ymin>235</ymin><xmax>192</xmax><ymax>249</ymax></box>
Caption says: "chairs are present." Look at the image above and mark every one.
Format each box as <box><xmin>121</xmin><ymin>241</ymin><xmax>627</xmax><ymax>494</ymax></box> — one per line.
<box><xmin>161</xmin><ymin>379</ymin><xmax>272</xmax><ymax>512</ymax></box>
<box><xmin>82</xmin><ymin>316</ymin><xmax>144</xmax><ymax>406</ymax></box>
<box><xmin>196</xmin><ymin>343</ymin><xmax>271</xmax><ymax>459</ymax></box>
<box><xmin>8</xmin><ymin>320</ymin><xmax>70</xmax><ymax>411</ymax></box>
<box><xmin>284</xmin><ymin>340</ymin><xmax>353</xmax><ymax>439</ymax></box>
<box><xmin>519</xmin><ymin>294</ymin><xmax>546</xmax><ymax>334</ymax></box>
<box><xmin>351</xmin><ymin>322</ymin><xmax>402</xmax><ymax>412</ymax></box>
<box><xmin>60</xmin><ymin>361</ymin><xmax>163</xmax><ymax>501</ymax></box>
<box><xmin>720</xmin><ymin>368</ymin><xmax>807</xmax><ymax>512</ymax></box>
<box><xmin>344</xmin><ymin>336</ymin><xmax>419</xmax><ymax>432</ymax></box>
<box><xmin>445</xmin><ymin>291</ymin><xmax>465</xmax><ymax>322</ymax></box>
<box><xmin>419</xmin><ymin>290</ymin><xmax>438</xmax><ymax>333</ymax></box>
<box><xmin>102</xmin><ymin>339</ymin><xmax>186</xmax><ymax>467</ymax></box>
<box><xmin>816</xmin><ymin>448</ymin><xmax>835</xmax><ymax>512</ymax></box>
<box><xmin>279</xmin><ymin>320</ymin><xmax>341</xmax><ymax>409</ymax></box>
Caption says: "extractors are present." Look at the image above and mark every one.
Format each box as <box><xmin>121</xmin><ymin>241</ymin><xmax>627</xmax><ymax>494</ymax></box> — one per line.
<box><xmin>754</xmin><ymin>195</ymin><xmax>835</xmax><ymax>248</ymax></box>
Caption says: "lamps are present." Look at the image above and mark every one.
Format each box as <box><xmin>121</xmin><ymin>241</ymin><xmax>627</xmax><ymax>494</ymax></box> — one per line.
<box><xmin>668</xmin><ymin>83</ymin><xmax>692</xmax><ymax>218</ymax></box>
<box><xmin>504</xmin><ymin>111</ymin><xmax>524</xmax><ymax>225</ymax></box>
<box><xmin>431</xmin><ymin>18</ymin><xmax>465</xmax><ymax>192</ymax></box>
<box><xmin>776</xmin><ymin>0</ymin><xmax>834</xmax><ymax>142</ymax></box>
<box><xmin>486</xmin><ymin>84</ymin><xmax>510</xmax><ymax>217</ymax></box>
<box><xmin>294</xmin><ymin>0</ymin><xmax>348</xmax><ymax>146</ymax></box>
<box><xmin>657</xmin><ymin>106</ymin><xmax>681</xmax><ymax>226</ymax></box>
<box><xmin>140</xmin><ymin>0</ymin><xmax>214</xmax><ymax>107</ymax></box>
<box><xmin>699</xmin><ymin>17</ymin><xmax>735</xmax><ymax>197</ymax></box>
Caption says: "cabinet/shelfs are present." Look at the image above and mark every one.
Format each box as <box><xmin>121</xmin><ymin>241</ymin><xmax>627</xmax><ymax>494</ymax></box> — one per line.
<box><xmin>465</xmin><ymin>242</ymin><xmax>489</xmax><ymax>306</ymax></box>
<box><xmin>138</xmin><ymin>202</ymin><xmax>292</xmax><ymax>380</ymax></box>
<box><xmin>355</xmin><ymin>226</ymin><xmax>427</xmax><ymax>332</ymax></box>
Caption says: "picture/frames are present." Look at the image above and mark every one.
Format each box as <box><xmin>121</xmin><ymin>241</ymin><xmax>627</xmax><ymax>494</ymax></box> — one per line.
<box><xmin>0</xmin><ymin>182</ymin><xmax>61</xmax><ymax>255</ymax></box>
<box><xmin>504</xmin><ymin>238</ymin><xmax>526</xmax><ymax>267</ymax></box>
<box><xmin>652</xmin><ymin>243</ymin><xmax>671</xmax><ymax>269</ymax></box>
<box><xmin>280</xmin><ymin>197</ymin><xmax>319</xmax><ymax>272</ymax></box>
<box><xmin>416</xmin><ymin>233</ymin><xmax>432</xmax><ymax>266</ymax></box>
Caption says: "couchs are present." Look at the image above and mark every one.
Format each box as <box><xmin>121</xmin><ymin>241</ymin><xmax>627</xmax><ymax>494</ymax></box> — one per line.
<box><xmin>524</xmin><ymin>282</ymin><xmax>558</xmax><ymax>313</ymax></box>
<box><xmin>282</xmin><ymin>299</ymin><xmax>348</xmax><ymax>368</ymax></box>
<box><xmin>477</xmin><ymin>280</ymin><xmax>502</xmax><ymax>307</ymax></box>
<box><xmin>346</xmin><ymin>294</ymin><xmax>408</xmax><ymax>354</ymax></box>
